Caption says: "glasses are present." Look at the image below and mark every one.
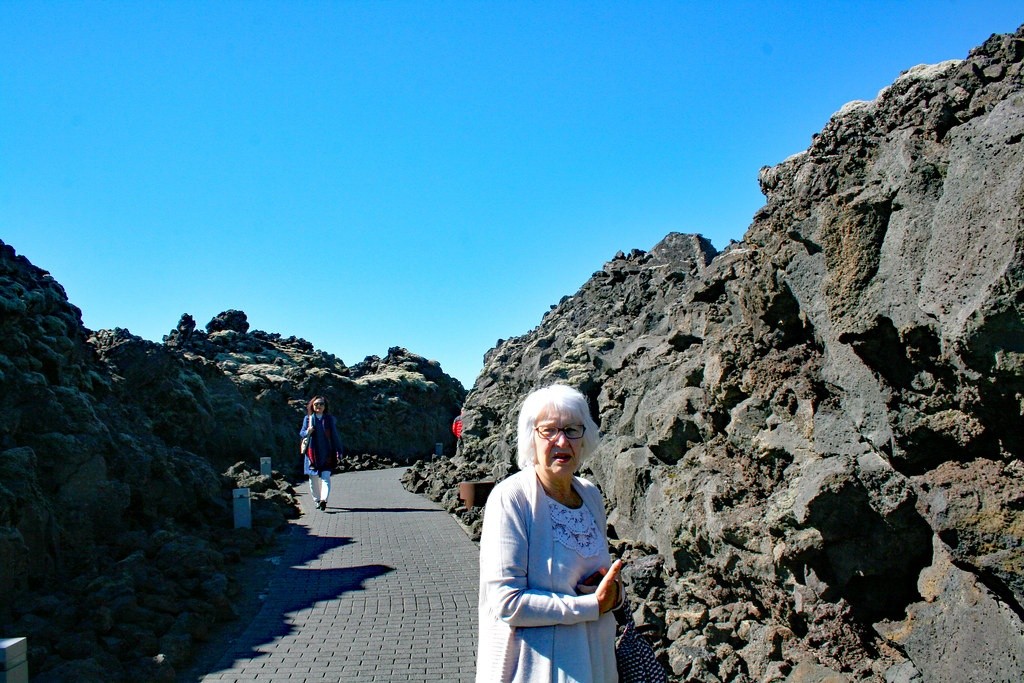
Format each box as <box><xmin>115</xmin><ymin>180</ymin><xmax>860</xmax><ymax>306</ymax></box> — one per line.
<box><xmin>313</xmin><ymin>402</ymin><xmax>325</xmax><ymax>406</ymax></box>
<box><xmin>533</xmin><ymin>424</ymin><xmax>587</xmax><ymax>439</ymax></box>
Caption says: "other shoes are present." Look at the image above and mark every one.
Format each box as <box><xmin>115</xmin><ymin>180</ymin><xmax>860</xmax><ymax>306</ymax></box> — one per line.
<box><xmin>315</xmin><ymin>501</ymin><xmax>320</xmax><ymax>509</ymax></box>
<box><xmin>319</xmin><ymin>500</ymin><xmax>326</xmax><ymax>511</ymax></box>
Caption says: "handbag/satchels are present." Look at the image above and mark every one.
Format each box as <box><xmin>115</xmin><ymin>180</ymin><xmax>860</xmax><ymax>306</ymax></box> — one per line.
<box><xmin>300</xmin><ymin>415</ymin><xmax>312</xmax><ymax>454</ymax></box>
<box><xmin>616</xmin><ymin>594</ymin><xmax>666</xmax><ymax>683</ymax></box>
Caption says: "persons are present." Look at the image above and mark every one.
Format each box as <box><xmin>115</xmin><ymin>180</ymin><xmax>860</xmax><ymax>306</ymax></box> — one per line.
<box><xmin>473</xmin><ymin>383</ymin><xmax>625</xmax><ymax>683</ymax></box>
<box><xmin>300</xmin><ymin>396</ymin><xmax>344</xmax><ymax>511</ymax></box>
<box><xmin>452</xmin><ymin>411</ymin><xmax>462</xmax><ymax>438</ymax></box>
